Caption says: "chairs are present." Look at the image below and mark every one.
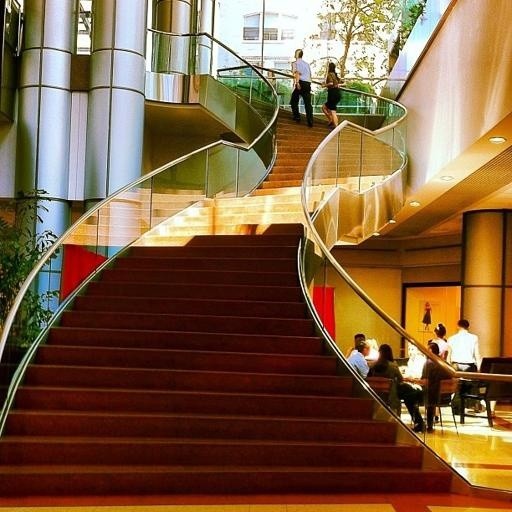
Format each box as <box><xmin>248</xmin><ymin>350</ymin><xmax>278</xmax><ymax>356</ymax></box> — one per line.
<box><xmin>357</xmin><ymin>356</ymin><xmax>511</xmax><ymax>436</ymax></box>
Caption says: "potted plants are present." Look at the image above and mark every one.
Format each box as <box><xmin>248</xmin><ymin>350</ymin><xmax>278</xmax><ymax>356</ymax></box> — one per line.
<box><xmin>0</xmin><ymin>188</ymin><xmax>63</xmax><ymax>407</ymax></box>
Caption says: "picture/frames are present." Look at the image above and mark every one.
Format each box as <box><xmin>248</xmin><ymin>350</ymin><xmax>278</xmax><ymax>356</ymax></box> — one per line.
<box><xmin>414</xmin><ymin>297</ymin><xmax>445</xmax><ymax>333</ymax></box>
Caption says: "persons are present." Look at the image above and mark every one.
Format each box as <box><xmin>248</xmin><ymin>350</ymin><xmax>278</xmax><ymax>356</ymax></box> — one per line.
<box><xmin>288</xmin><ymin>48</ymin><xmax>315</xmax><ymax>129</ymax></box>
<box><xmin>344</xmin><ymin>341</ymin><xmax>370</xmax><ymax>378</ymax></box>
<box><xmin>346</xmin><ymin>333</ymin><xmax>379</xmax><ymax>360</ymax></box>
<box><xmin>404</xmin><ymin>342</ymin><xmax>428</xmax><ymax>389</ymax></box>
<box><xmin>401</xmin><ymin>343</ymin><xmax>453</xmax><ymax>434</ymax></box>
<box><xmin>430</xmin><ymin>322</ymin><xmax>448</xmax><ymax>362</ymax></box>
<box><xmin>421</xmin><ymin>301</ymin><xmax>433</xmax><ymax>331</ymax></box>
<box><xmin>366</xmin><ymin>344</ymin><xmax>404</xmax><ymax>417</ymax></box>
<box><xmin>320</xmin><ymin>62</ymin><xmax>344</xmax><ymax>129</ymax></box>
<box><xmin>447</xmin><ymin>319</ymin><xmax>484</xmax><ymax>414</ymax></box>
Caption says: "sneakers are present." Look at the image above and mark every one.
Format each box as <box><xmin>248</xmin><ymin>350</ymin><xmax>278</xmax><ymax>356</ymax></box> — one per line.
<box><xmin>411</xmin><ymin>423</ymin><xmax>426</xmax><ymax>433</ymax></box>
<box><xmin>427</xmin><ymin>424</ymin><xmax>434</xmax><ymax>433</ymax></box>
<box><xmin>327</xmin><ymin>118</ymin><xmax>337</xmax><ymax>132</ymax></box>
<box><xmin>294</xmin><ymin>117</ymin><xmax>313</xmax><ymax>127</ymax></box>
